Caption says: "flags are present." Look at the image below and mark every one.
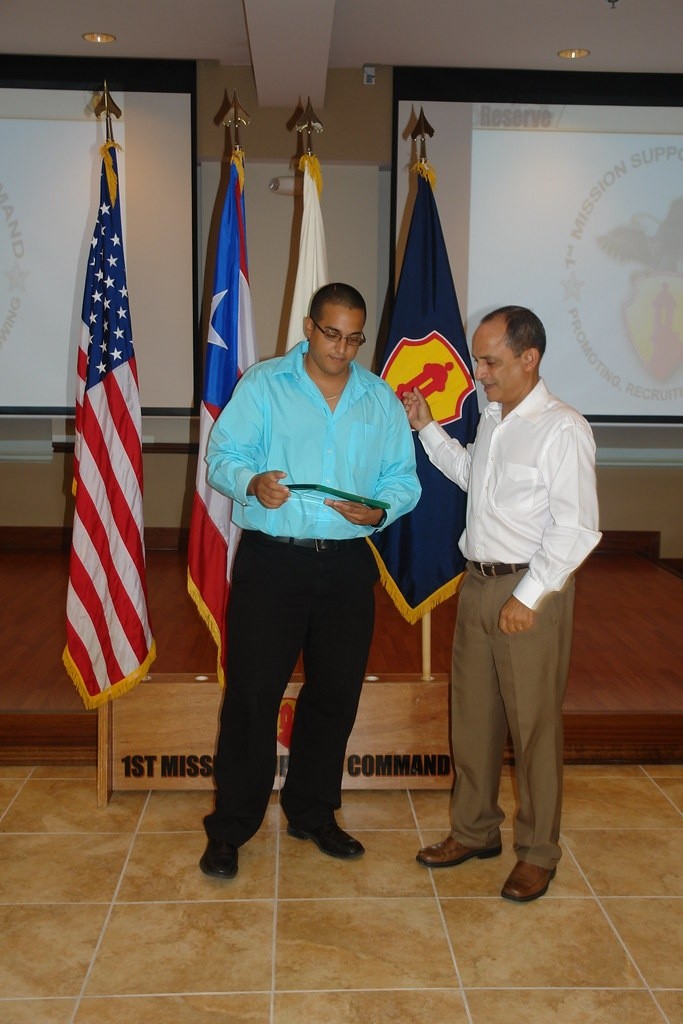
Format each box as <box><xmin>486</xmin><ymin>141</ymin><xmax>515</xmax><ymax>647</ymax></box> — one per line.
<box><xmin>187</xmin><ymin>148</ymin><xmax>257</xmax><ymax>690</ymax></box>
<box><xmin>284</xmin><ymin>156</ymin><xmax>326</xmax><ymax>358</ymax></box>
<box><xmin>365</xmin><ymin>165</ymin><xmax>479</xmax><ymax>625</ymax></box>
<box><xmin>60</xmin><ymin>140</ymin><xmax>158</xmax><ymax>711</ymax></box>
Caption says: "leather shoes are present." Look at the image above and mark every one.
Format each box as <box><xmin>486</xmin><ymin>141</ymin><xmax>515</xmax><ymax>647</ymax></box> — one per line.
<box><xmin>415</xmin><ymin>835</ymin><xmax>502</xmax><ymax>867</ymax></box>
<box><xmin>501</xmin><ymin>860</ymin><xmax>556</xmax><ymax>902</ymax></box>
<box><xmin>199</xmin><ymin>839</ymin><xmax>239</xmax><ymax>878</ymax></box>
<box><xmin>287</xmin><ymin>809</ymin><xmax>365</xmax><ymax>859</ymax></box>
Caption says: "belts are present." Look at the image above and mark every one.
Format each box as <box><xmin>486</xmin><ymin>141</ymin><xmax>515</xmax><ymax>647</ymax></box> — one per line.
<box><xmin>242</xmin><ymin>530</ymin><xmax>365</xmax><ymax>553</ymax></box>
<box><xmin>473</xmin><ymin>561</ymin><xmax>529</xmax><ymax>577</ymax></box>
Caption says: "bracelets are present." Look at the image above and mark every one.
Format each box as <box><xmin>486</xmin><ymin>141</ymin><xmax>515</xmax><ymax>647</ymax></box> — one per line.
<box><xmin>371</xmin><ymin>509</ymin><xmax>387</xmax><ymax>528</ymax></box>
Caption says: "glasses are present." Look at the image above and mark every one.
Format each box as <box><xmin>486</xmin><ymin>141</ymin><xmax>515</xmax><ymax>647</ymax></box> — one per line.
<box><xmin>309</xmin><ymin>315</ymin><xmax>366</xmax><ymax>347</ymax></box>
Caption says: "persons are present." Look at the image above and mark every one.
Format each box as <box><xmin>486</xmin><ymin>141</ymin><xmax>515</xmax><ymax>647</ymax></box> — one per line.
<box><xmin>197</xmin><ymin>282</ymin><xmax>422</xmax><ymax>882</ymax></box>
<box><xmin>400</xmin><ymin>305</ymin><xmax>603</xmax><ymax>903</ymax></box>
